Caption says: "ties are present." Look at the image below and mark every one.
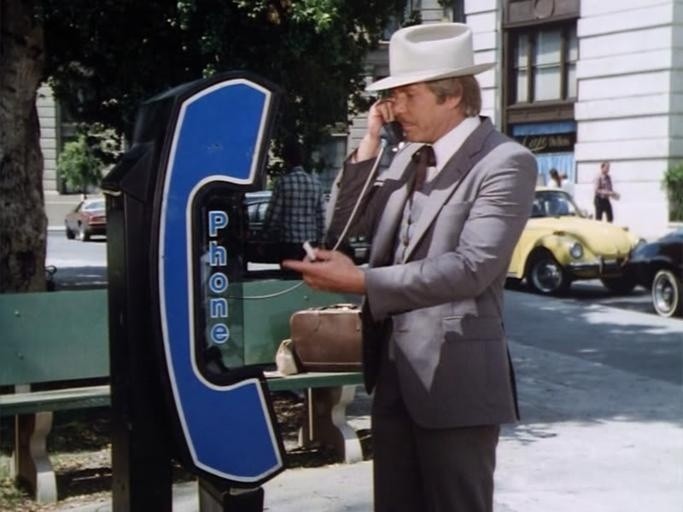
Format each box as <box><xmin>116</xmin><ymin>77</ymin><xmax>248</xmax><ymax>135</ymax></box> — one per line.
<box><xmin>393</xmin><ymin>146</ymin><xmax>436</xmax><ymax>268</ymax></box>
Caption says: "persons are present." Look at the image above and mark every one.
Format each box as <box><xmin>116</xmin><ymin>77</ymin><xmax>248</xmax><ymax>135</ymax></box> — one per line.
<box><xmin>240</xmin><ymin>194</ymin><xmax>254</xmax><ymax>278</ymax></box>
<box><xmin>547</xmin><ymin>168</ymin><xmax>560</xmax><ymax>188</ymax></box>
<box><xmin>558</xmin><ymin>170</ymin><xmax>572</xmax><ymax>214</ymax></box>
<box><xmin>593</xmin><ymin>162</ymin><xmax>619</xmax><ymax>223</ymax></box>
<box><xmin>280</xmin><ymin>21</ymin><xmax>537</xmax><ymax>511</ymax></box>
<box><xmin>258</xmin><ymin>140</ymin><xmax>324</xmax><ymax>280</ymax></box>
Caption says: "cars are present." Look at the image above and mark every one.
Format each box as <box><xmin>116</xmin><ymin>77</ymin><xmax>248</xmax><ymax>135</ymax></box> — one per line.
<box><xmin>506</xmin><ymin>187</ymin><xmax>641</xmax><ymax>297</ymax></box>
<box><xmin>63</xmin><ymin>197</ymin><xmax>106</xmax><ymax>243</ymax></box>
<box><xmin>247</xmin><ymin>189</ymin><xmax>372</xmax><ymax>265</ymax></box>
<box><xmin>624</xmin><ymin>229</ymin><xmax>683</xmax><ymax>319</ymax></box>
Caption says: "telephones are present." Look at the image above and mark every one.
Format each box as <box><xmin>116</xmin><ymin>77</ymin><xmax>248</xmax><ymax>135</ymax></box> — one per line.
<box><xmin>377</xmin><ymin>89</ymin><xmax>405</xmax><ymax>144</ymax></box>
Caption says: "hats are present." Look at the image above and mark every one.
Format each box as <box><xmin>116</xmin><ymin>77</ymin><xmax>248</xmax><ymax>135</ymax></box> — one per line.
<box><xmin>364</xmin><ymin>22</ymin><xmax>496</xmax><ymax>91</ymax></box>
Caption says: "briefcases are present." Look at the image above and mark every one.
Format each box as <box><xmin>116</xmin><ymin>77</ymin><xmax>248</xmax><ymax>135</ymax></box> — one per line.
<box><xmin>291</xmin><ymin>304</ymin><xmax>366</xmax><ymax>371</ymax></box>
